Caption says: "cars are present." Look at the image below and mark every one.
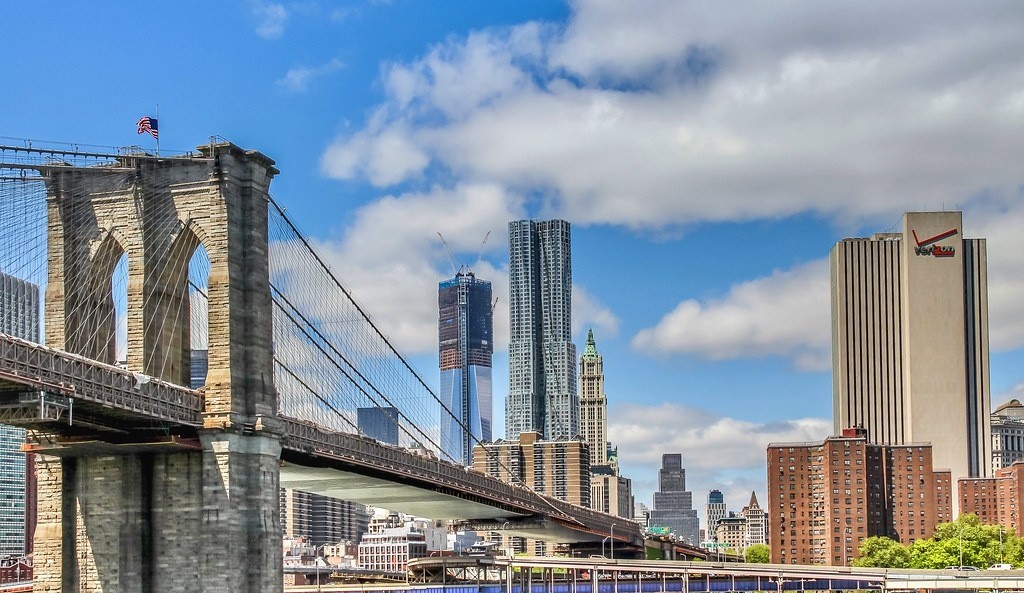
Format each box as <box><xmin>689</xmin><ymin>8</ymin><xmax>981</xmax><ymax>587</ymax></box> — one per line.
<box><xmin>590</xmin><ymin>554</ymin><xmax>606</xmax><ymax>558</ymax></box>
<box><xmin>958</xmin><ymin>565</ymin><xmax>979</xmax><ymax>571</ymax></box>
<box><xmin>943</xmin><ymin>566</ymin><xmax>959</xmax><ymax>569</ymax></box>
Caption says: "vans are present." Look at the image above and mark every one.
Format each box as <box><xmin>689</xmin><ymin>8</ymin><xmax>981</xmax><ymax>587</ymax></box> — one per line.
<box><xmin>987</xmin><ymin>564</ymin><xmax>1011</xmax><ymax>570</ymax></box>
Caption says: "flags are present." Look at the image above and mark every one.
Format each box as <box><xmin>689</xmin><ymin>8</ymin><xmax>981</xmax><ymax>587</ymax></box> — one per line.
<box><xmin>136</xmin><ymin>116</ymin><xmax>158</xmax><ymax>139</ymax></box>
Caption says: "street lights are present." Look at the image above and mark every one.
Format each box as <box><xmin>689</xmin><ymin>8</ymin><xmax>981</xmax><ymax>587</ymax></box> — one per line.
<box><xmin>680</xmin><ymin>554</ymin><xmax>686</xmax><ymax>561</ymax></box>
<box><xmin>503</xmin><ymin>522</ymin><xmax>510</xmax><ymax>556</ymax></box>
<box><xmin>450</xmin><ymin>539</ymin><xmax>461</xmax><ymax>555</ymax></box>
<box><xmin>998</xmin><ymin>527</ymin><xmax>1003</xmax><ymax>563</ymax></box>
<box><xmin>602</xmin><ymin>536</ymin><xmax>610</xmax><ymax>557</ymax></box>
<box><xmin>960</xmin><ymin>530</ymin><xmax>964</xmax><ymax>565</ymax></box>
<box><xmin>316</xmin><ymin>543</ymin><xmax>329</xmax><ymax>591</ymax></box>
<box><xmin>611</xmin><ymin>523</ymin><xmax>616</xmax><ymax>559</ymax></box>
<box><xmin>844</xmin><ymin>528</ymin><xmax>848</xmax><ymax>566</ymax></box>
